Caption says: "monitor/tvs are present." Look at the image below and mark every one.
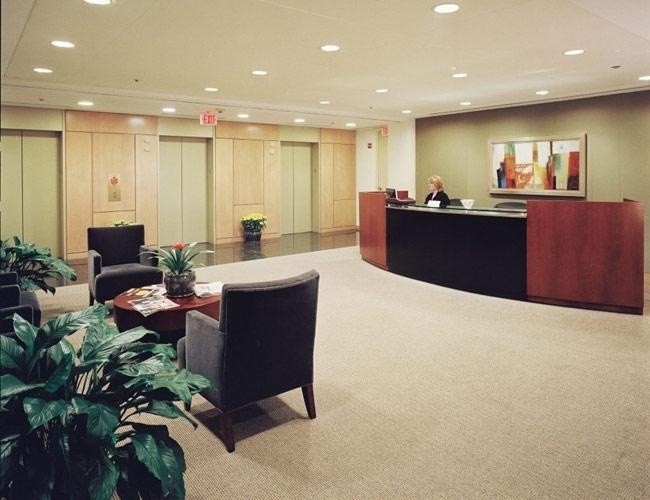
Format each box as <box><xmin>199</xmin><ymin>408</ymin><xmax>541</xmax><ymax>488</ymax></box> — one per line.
<box><xmin>385</xmin><ymin>187</ymin><xmax>396</xmax><ymax>198</ymax></box>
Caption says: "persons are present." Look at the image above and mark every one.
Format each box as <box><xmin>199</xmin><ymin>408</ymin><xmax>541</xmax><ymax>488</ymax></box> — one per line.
<box><xmin>424</xmin><ymin>175</ymin><xmax>450</xmax><ymax>208</ymax></box>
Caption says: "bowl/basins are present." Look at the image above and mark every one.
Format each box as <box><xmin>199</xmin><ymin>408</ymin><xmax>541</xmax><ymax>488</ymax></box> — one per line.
<box><xmin>459</xmin><ymin>199</ymin><xmax>475</xmax><ymax>209</ymax></box>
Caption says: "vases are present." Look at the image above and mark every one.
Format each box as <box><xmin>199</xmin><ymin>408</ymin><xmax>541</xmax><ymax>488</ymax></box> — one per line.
<box><xmin>244</xmin><ymin>228</ymin><xmax>261</xmax><ymax>246</ymax></box>
<box><xmin>164</xmin><ymin>269</ymin><xmax>197</xmax><ymax>298</ymax></box>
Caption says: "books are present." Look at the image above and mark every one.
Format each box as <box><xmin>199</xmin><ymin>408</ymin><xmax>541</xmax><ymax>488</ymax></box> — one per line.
<box><xmin>194</xmin><ymin>281</ymin><xmax>223</xmax><ymax>298</ymax></box>
<box><xmin>125</xmin><ymin>284</ymin><xmax>181</xmax><ymax>319</ymax></box>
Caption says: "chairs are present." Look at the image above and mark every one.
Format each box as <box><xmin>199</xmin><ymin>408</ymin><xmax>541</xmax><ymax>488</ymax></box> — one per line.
<box><xmin>494</xmin><ymin>202</ymin><xmax>527</xmax><ymax>209</ymax></box>
<box><xmin>86</xmin><ymin>224</ymin><xmax>163</xmax><ymax>315</ymax></box>
<box><xmin>0</xmin><ymin>270</ymin><xmax>42</xmax><ymax>337</ymax></box>
<box><xmin>447</xmin><ymin>198</ymin><xmax>463</xmax><ymax>206</ymax></box>
<box><xmin>177</xmin><ymin>269</ymin><xmax>319</xmax><ymax>453</ymax></box>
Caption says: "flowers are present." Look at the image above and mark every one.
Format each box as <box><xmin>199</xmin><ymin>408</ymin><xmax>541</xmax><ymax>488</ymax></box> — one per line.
<box><xmin>240</xmin><ymin>213</ymin><xmax>268</xmax><ymax>231</ymax></box>
<box><xmin>137</xmin><ymin>240</ymin><xmax>215</xmax><ymax>276</ymax></box>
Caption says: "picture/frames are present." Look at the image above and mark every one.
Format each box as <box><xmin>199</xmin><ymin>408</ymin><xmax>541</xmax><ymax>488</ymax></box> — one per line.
<box><xmin>487</xmin><ymin>133</ymin><xmax>587</xmax><ymax>198</ymax></box>
